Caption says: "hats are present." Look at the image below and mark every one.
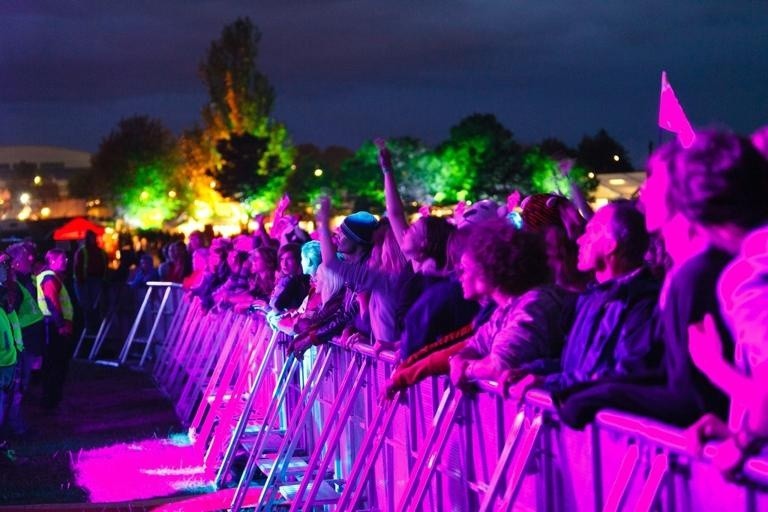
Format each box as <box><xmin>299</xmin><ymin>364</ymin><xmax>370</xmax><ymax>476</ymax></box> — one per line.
<box><xmin>341</xmin><ymin>210</ymin><xmax>378</xmax><ymax>246</ymax></box>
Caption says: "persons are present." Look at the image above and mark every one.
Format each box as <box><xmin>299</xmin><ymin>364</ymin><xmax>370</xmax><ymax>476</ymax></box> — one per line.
<box><xmin>1</xmin><ymin>124</ymin><xmax>766</xmax><ymax>484</ymax></box>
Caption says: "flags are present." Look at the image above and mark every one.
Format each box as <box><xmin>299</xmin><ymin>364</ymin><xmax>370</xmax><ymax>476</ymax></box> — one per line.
<box><xmin>658</xmin><ymin>70</ymin><xmax>696</xmax><ymax>148</ymax></box>
